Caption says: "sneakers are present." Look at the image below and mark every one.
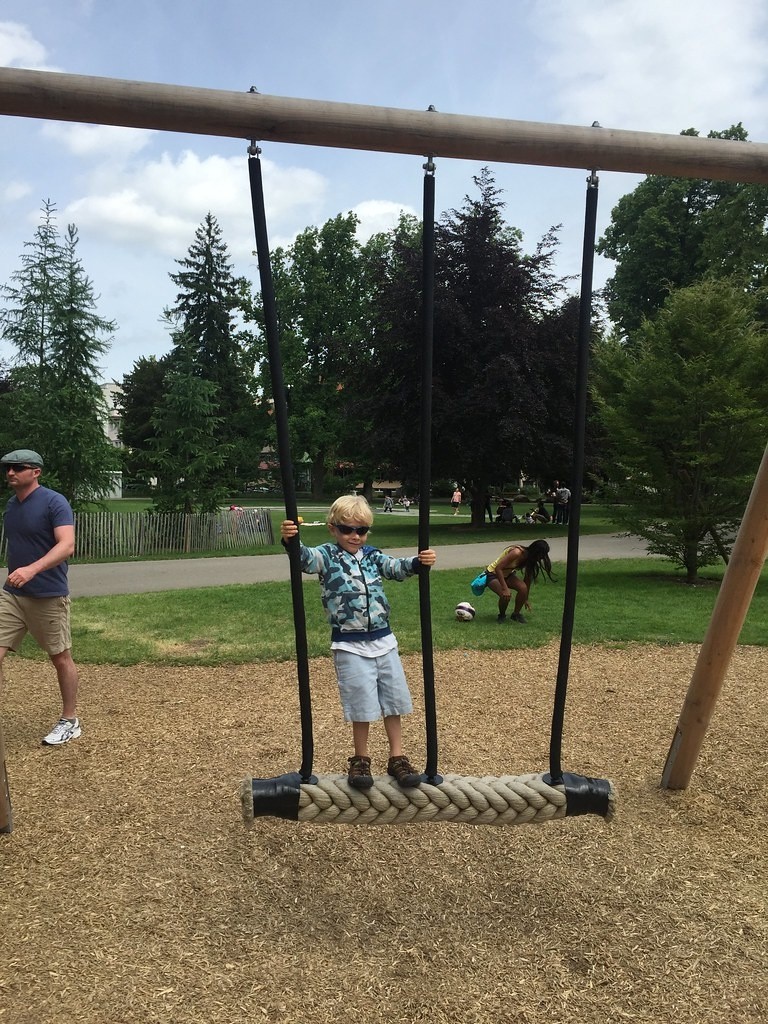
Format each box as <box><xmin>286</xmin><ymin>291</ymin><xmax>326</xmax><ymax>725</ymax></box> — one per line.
<box><xmin>42</xmin><ymin>716</ymin><xmax>82</xmax><ymax>745</ymax></box>
<box><xmin>387</xmin><ymin>756</ymin><xmax>421</xmax><ymax>787</ymax></box>
<box><xmin>347</xmin><ymin>756</ymin><xmax>374</xmax><ymax>788</ymax></box>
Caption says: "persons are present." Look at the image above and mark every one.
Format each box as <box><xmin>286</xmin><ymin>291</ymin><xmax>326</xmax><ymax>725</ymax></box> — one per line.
<box><xmin>524</xmin><ymin>499</ymin><xmax>550</xmax><ymax>524</ymax></box>
<box><xmin>0</xmin><ymin>449</ymin><xmax>82</xmax><ymax>746</ymax></box>
<box><xmin>237</xmin><ymin>505</ymin><xmax>244</xmax><ymax>516</ymax></box>
<box><xmin>226</xmin><ymin>505</ymin><xmax>235</xmax><ymax>512</ymax></box>
<box><xmin>398</xmin><ymin>495</ymin><xmax>420</xmax><ymax>512</ymax></box>
<box><xmin>550</xmin><ymin>480</ymin><xmax>572</xmax><ymax>526</ymax></box>
<box><xmin>383</xmin><ymin>495</ymin><xmax>393</xmax><ymax>512</ymax></box>
<box><xmin>486</xmin><ymin>540</ymin><xmax>559</xmax><ymax>624</ymax></box>
<box><xmin>297</xmin><ymin>514</ymin><xmax>304</xmax><ymax>524</ymax></box>
<box><xmin>280</xmin><ymin>495</ymin><xmax>437</xmax><ymax>788</ymax></box>
<box><xmin>450</xmin><ymin>488</ymin><xmax>462</xmax><ymax>516</ymax></box>
<box><xmin>215</xmin><ymin>508</ymin><xmax>268</xmax><ymax>535</ymax></box>
<box><xmin>495</xmin><ymin>498</ymin><xmax>514</xmax><ymax>524</ymax></box>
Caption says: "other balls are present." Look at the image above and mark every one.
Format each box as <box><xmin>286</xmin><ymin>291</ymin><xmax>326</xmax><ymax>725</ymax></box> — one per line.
<box><xmin>455</xmin><ymin>601</ymin><xmax>476</xmax><ymax>621</ymax></box>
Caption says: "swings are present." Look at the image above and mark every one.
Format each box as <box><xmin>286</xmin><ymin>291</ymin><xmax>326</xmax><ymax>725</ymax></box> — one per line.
<box><xmin>242</xmin><ymin>140</ymin><xmax>618</xmax><ymax>824</ymax></box>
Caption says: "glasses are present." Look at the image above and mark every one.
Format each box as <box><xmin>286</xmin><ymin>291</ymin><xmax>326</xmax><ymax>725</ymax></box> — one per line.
<box><xmin>5</xmin><ymin>465</ymin><xmax>38</xmax><ymax>472</ymax></box>
<box><xmin>332</xmin><ymin>524</ymin><xmax>370</xmax><ymax>536</ymax></box>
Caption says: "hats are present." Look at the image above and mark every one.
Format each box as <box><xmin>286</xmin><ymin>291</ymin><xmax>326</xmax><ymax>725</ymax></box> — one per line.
<box><xmin>0</xmin><ymin>449</ymin><xmax>43</xmax><ymax>466</ymax></box>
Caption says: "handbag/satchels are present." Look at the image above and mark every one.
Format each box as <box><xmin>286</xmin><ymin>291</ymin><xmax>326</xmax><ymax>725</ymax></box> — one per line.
<box><xmin>470</xmin><ymin>572</ymin><xmax>488</xmax><ymax>596</ymax></box>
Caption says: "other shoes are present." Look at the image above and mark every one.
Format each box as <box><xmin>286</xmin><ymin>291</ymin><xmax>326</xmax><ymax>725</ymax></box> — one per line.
<box><xmin>498</xmin><ymin>614</ymin><xmax>506</xmax><ymax>624</ymax></box>
<box><xmin>510</xmin><ymin>612</ymin><xmax>527</xmax><ymax>623</ymax></box>
